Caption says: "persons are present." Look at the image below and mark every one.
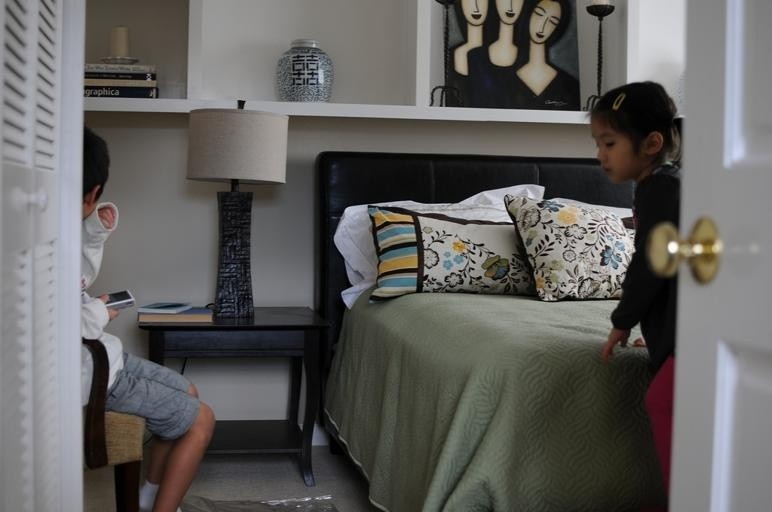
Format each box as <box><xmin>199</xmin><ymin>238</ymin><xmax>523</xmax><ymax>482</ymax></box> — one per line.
<box><xmin>512</xmin><ymin>0</ymin><xmax>579</xmax><ymax>109</ymax></box>
<box><xmin>82</xmin><ymin>126</ymin><xmax>216</xmax><ymax>512</ymax></box>
<box><xmin>589</xmin><ymin>80</ymin><xmax>684</xmax><ymax>499</ymax></box>
<box><xmin>466</xmin><ymin>0</ymin><xmax>529</xmax><ymax>109</ymax></box>
<box><xmin>444</xmin><ymin>0</ymin><xmax>498</xmax><ymax>107</ymax></box>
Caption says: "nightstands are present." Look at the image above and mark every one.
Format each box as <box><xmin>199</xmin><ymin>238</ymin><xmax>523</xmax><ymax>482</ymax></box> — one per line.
<box><xmin>135</xmin><ymin>304</ymin><xmax>330</xmax><ymax>489</ymax></box>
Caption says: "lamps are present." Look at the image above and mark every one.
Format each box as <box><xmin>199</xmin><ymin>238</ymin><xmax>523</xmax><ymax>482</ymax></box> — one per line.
<box><xmin>583</xmin><ymin>3</ymin><xmax>620</xmax><ymax>115</ymax></box>
<box><xmin>186</xmin><ymin>100</ymin><xmax>292</xmax><ymax>320</ymax></box>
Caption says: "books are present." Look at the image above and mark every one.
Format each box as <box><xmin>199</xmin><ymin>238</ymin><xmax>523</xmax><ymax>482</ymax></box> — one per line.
<box><xmin>137</xmin><ymin>302</ymin><xmax>193</xmax><ymax>314</ymax></box>
<box><xmin>136</xmin><ymin>308</ymin><xmax>215</xmax><ymax>323</ymax></box>
<box><xmin>84</xmin><ymin>62</ymin><xmax>159</xmax><ymax>99</ymax></box>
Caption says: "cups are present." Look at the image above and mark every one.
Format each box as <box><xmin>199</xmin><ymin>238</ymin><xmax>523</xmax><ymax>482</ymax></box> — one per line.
<box><xmin>110</xmin><ymin>26</ymin><xmax>131</xmax><ymax>57</ymax></box>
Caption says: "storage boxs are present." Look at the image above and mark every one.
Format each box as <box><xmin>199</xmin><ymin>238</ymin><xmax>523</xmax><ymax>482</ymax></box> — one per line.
<box><xmin>84</xmin><ymin>59</ymin><xmax>163</xmax><ymax>101</ymax></box>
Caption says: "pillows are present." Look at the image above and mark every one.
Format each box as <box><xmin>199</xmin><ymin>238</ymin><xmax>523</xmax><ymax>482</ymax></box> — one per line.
<box><xmin>501</xmin><ymin>192</ymin><xmax>635</xmax><ymax>304</ymax></box>
<box><xmin>367</xmin><ymin>204</ymin><xmax>536</xmax><ymax>300</ymax></box>
<box><xmin>332</xmin><ymin>179</ymin><xmax>548</xmax><ymax>310</ymax></box>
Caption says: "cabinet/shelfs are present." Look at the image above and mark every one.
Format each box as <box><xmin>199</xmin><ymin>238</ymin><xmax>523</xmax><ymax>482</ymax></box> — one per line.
<box><xmin>85</xmin><ymin>2</ymin><xmax>637</xmax><ymax>126</ymax></box>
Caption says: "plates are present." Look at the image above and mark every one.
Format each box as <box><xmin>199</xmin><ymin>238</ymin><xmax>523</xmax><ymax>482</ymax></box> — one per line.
<box><xmin>101</xmin><ymin>56</ymin><xmax>140</xmax><ymax>67</ymax></box>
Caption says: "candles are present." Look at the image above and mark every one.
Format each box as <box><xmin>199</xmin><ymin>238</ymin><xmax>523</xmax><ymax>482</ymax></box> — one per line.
<box><xmin>107</xmin><ymin>24</ymin><xmax>131</xmax><ymax>58</ymax></box>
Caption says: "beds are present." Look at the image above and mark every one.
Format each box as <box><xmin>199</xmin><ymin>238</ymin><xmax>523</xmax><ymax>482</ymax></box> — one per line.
<box><xmin>317</xmin><ymin>143</ymin><xmax>672</xmax><ymax>512</ymax></box>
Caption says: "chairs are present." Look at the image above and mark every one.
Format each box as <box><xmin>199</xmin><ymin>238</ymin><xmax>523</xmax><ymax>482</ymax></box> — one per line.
<box><xmin>82</xmin><ymin>336</ymin><xmax>147</xmax><ymax>511</ymax></box>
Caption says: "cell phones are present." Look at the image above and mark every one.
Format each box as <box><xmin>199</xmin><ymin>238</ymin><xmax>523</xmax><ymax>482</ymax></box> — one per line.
<box><xmin>96</xmin><ymin>289</ymin><xmax>136</xmax><ymax>309</ymax></box>
